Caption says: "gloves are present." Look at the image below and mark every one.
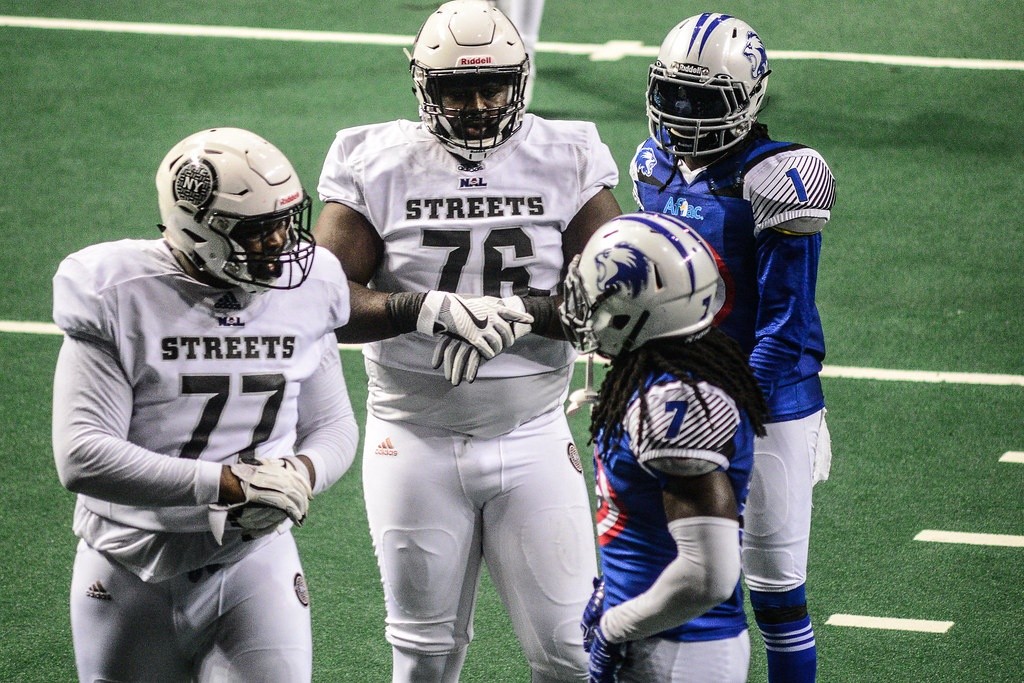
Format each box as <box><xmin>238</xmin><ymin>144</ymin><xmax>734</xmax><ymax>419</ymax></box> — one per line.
<box><xmin>580</xmin><ymin>578</ymin><xmax>618</xmax><ymax>683</ymax></box>
<box><xmin>208</xmin><ymin>455</ymin><xmax>314</xmax><ymax>539</ymax></box>
<box><xmin>416</xmin><ymin>290</ymin><xmax>534</xmax><ymax>386</ymax></box>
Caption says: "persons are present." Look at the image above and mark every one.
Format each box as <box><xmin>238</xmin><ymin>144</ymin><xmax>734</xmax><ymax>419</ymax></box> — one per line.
<box><xmin>558</xmin><ymin>211</ymin><xmax>767</xmax><ymax>683</ymax></box>
<box><xmin>310</xmin><ymin>0</ymin><xmax>622</xmax><ymax>683</ymax></box>
<box><xmin>627</xmin><ymin>13</ymin><xmax>837</xmax><ymax>683</ymax></box>
<box><xmin>50</xmin><ymin>127</ymin><xmax>360</xmax><ymax>683</ymax></box>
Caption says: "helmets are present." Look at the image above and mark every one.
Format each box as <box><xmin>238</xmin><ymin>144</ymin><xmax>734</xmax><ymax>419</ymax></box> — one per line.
<box><xmin>559</xmin><ymin>212</ymin><xmax>730</xmax><ymax>359</ymax></box>
<box><xmin>410</xmin><ymin>0</ymin><xmax>530</xmax><ymax>161</ymax></box>
<box><xmin>646</xmin><ymin>12</ymin><xmax>770</xmax><ymax>159</ymax></box>
<box><xmin>156</xmin><ymin>128</ymin><xmax>316</xmax><ymax>294</ymax></box>
